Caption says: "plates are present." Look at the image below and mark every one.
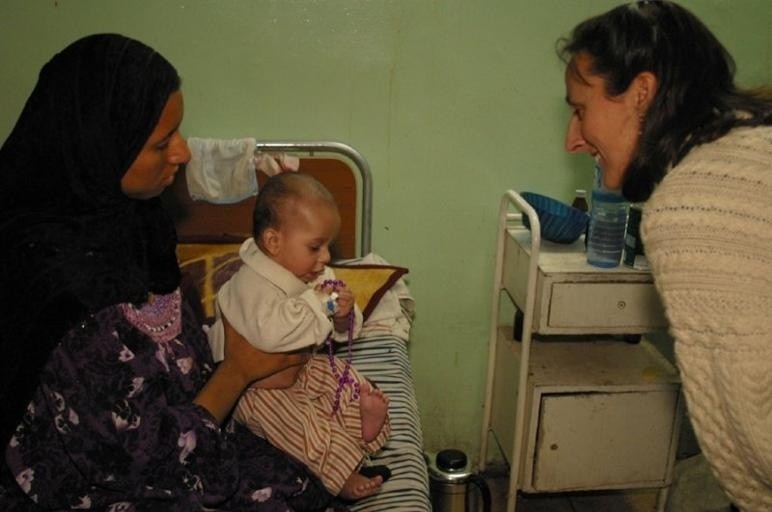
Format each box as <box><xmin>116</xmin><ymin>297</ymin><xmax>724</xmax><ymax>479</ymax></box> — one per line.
<box><xmin>479</xmin><ymin>189</ymin><xmax>685</xmax><ymax>512</ymax></box>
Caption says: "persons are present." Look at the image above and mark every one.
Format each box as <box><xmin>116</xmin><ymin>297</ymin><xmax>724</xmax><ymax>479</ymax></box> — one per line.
<box><xmin>207</xmin><ymin>171</ymin><xmax>391</xmax><ymax>501</ymax></box>
<box><xmin>0</xmin><ymin>30</ymin><xmax>345</xmax><ymax>512</ymax></box>
<box><xmin>554</xmin><ymin>0</ymin><xmax>772</xmax><ymax>511</ymax></box>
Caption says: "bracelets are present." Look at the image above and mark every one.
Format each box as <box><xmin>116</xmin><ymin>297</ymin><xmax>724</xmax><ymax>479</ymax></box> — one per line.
<box><xmin>520</xmin><ymin>191</ymin><xmax>588</xmax><ymax>245</ymax></box>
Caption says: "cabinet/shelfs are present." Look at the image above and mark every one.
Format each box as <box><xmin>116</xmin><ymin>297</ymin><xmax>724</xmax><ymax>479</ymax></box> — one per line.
<box><xmin>587</xmin><ymin>165</ymin><xmax>626</xmax><ymax>268</ymax></box>
<box><xmin>572</xmin><ymin>190</ymin><xmax>588</xmax><ymax>213</ymax></box>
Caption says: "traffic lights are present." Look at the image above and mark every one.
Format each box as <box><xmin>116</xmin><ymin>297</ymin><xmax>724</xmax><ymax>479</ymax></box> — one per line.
<box><xmin>177</xmin><ymin>238</ymin><xmax>408</xmax><ymax>355</ymax></box>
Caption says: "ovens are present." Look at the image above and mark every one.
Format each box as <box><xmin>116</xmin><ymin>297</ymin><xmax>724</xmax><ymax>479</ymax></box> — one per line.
<box><xmin>0</xmin><ymin>139</ymin><xmax>433</xmax><ymax>512</ymax></box>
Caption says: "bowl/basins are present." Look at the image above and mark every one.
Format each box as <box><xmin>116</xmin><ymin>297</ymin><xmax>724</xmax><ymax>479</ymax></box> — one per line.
<box><xmin>315</xmin><ymin>279</ymin><xmax>360</xmax><ymax>418</ymax></box>
